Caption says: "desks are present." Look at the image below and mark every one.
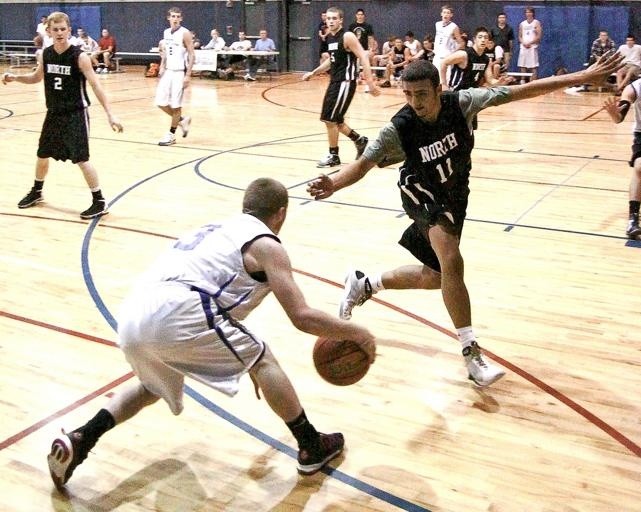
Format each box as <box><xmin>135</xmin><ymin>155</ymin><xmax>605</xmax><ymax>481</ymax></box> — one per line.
<box><xmin>196</xmin><ymin>49</ymin><xmax>280</xmax><ymax>82</ymax></box>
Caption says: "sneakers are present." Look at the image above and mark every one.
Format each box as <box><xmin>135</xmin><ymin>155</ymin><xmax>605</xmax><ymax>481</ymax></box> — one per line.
<box><xmin>47</xmin><ymin>429</ymin><xmax>88</xmax><ymax>491</ymax></box>
<box><xmin>158</xmin><ymin>131</ymin><xmax>177</xmax><ymax>145</ymax></box>
<box><xmin>177</xmin><ymin>114</ymin><xmax>192</xmax><ymax>137</ymax></box>
<box><xmin>355</xmin><ymin>135</ymin><xmax>369</xmax><ymax>159</ymax></box>
<box><xmin>95</xmin><ymin>64</ymin><xmax>112</xmax><ymax>75</ymax></box>
<box><xmin>338</xmin><ymin>268</ymin><xmax>375</xmax><ymax>320</ymax></box>
<box><xmin>201</xmin><ymin>68</ymin><xmax>257</xmax><ymax>81</ymax></box>
<box><xmin>355</xmin><ymin>72</ymin><xmax>392</xmax><ymax>93</ymax></box>
<box><xmin>462</xmin><ymin>341</ymin><xmax>507</xmax><ymax>389</ymax></box>
<box><xmin>79</xmin><ymin>197</ymin><xmax>109</xmax><ymax>219</ymax></box>
<box><xmin>626</xmin><ymin>214</ymin><xmax>641</xmax><ymax>239</ymax></box>
<box><xmin>317</xmin><ymin>154</ymin><xmax>341</xmax><ymax>167</ymax></box>
<box><xmin>296</xmin><ymin>433</ymin><xmax>345</xmax><ymax>477</ymax></box>
<box><xmin>17</xmin><ymin>187</ymin><xmax>43</xmax><ymax>210</ymax></box>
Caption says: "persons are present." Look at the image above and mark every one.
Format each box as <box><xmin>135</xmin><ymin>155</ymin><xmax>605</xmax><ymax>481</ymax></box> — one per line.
<box><xmin>578</xmin><ymin>31</ymin><xmax>617</xmax><ymax>92</ymax></box>
<box><xmin>49</xmin><ymin>179</ymin><xmax>379</xmax><ymax>490</ymax></box>
<box><xmin>319</xmin><ymin>4</ymin><xmax>542</xmax><ymax>128</ymax></box>
<box><xmin>602</xmin><ymin>78</ymin><xmax>641</xmax><ymax>238</ymax></box>
<box><xmin>613</xmin><ymin>35</ymin><xmax>641</xmax><ymax>95</ymax></box>
<box><xmin>300</xmin><ymin>6</ymin><xmax>383</xmax><ymax>169</ymax></box>
<box><xmin>307</xmin><ymin>50</ymin><xmax>627</xmax><ymax>388</ymax></box>
<box><xmin>190</xmin><ymin>29</ymin><xmax>276</xmax><ymax>80</ymax></box>
<box><xmin>34</xmin><ymin>16</ymin><xmax>116</xmax><ymax>74</ymax></box>
<box><xmin>154</xmin><ymin>6</ymin><xmax>195</xmax><ymax>146</ymax></box>
<box><xmin>2</xmin><ymin>12</ymin><xmax>125</xmax><ymax>218</ymax></box>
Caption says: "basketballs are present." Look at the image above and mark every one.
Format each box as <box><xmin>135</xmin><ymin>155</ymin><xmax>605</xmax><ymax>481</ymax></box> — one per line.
<box><xmin>314</xmin><ymin>335</ymin><xmax>369</xmax><ymax>386</ymax></box>
<box><xmin>110</xmin><ymin>64</ymin><xmax>116</xmax><ymax>70</ymax></box>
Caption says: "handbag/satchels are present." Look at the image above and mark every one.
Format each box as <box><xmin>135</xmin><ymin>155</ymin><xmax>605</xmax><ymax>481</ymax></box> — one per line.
<box><xmin>144</xmin><ymin>62</ymin><xmax>159</xmax><ymax>77</ymax></box>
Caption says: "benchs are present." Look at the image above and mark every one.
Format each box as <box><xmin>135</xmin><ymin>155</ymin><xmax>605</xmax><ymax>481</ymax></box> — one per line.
<box><xmin>354</xmin><ymin>64</ymin><xmax>392</xmax><ymax>77</ymax></box>
<box><xmin>105</xmin><ymin>56</ymin><xmax>124</xmax><ymax>74</ymax></box>
<box><xmin>508</xmin><ymin>70</ymin><xmax>541</xmax><ymax>82</ymax></box>
<box><xmin>112</xmin><ymin>49</ymin><xmax>163</xmax><ymax>58</ymax></box>
<box><xmin>3</xmin><ymin>51</ymin><xmax>42</xmax><ymax>68</ymax></box>
